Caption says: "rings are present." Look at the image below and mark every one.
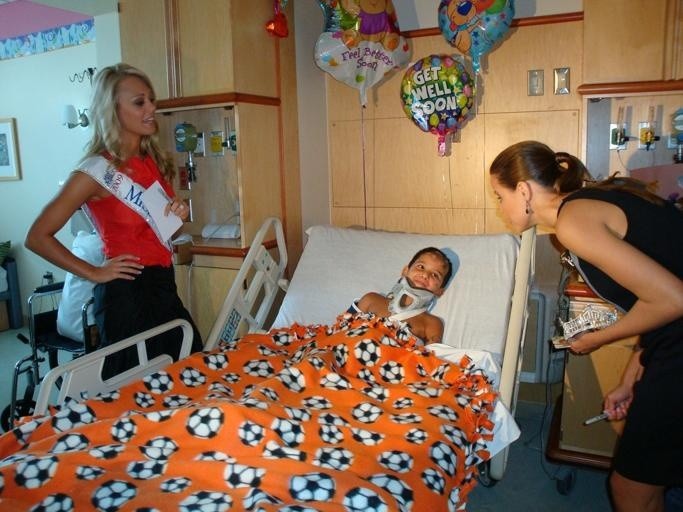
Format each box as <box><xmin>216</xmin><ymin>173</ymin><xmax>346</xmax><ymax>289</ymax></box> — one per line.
<box><xmin>179</xmin><ymin>204</ymin><xmax>185</xmax><ymax>209</ymax></box>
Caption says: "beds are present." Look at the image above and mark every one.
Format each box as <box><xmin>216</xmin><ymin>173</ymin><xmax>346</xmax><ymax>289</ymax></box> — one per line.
<box><xmin>1</xmin><ymin>216</ymin><xmax>536</xmax><ymax>511</ymax></box>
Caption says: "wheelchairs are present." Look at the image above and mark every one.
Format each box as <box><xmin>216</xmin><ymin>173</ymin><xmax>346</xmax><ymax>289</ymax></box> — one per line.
<box><xmin>0</xmin><ymin>271</ymin><xmax>101</xmax><ymax>432</ymax></box>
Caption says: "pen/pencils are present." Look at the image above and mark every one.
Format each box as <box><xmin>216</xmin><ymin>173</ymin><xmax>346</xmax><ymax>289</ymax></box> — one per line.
<box><xmin>159</xmin><ymin>188</ymin><xmax>172</xmax><ymax>206</ymax></box>
<box><xmin>583</xmin><ymin>400</ymin><xmax>627</xmax><ymax>426</ymax></box>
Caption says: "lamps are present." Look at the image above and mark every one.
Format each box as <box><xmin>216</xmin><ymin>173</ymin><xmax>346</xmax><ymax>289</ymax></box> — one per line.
<box><xmin>61</xmin><ymin>103</ymin><xmax>91</xmax><ymax>128</ymax></box>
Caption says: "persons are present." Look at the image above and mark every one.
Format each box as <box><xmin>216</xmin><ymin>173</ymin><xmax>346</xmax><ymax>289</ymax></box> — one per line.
<box><xmin>345</xmin><ymin>247</ymin><xmax>452</xmax><ymax>345</ymax></box>
<box><xmin>489</xmin><ymin>140</ymin><xmax>683</xmax><ymax>512</ymax></box>
<box><xmin>24</xmin><ymin>63</ymin><xmax>205</xmax><ymax>380</ymax></box>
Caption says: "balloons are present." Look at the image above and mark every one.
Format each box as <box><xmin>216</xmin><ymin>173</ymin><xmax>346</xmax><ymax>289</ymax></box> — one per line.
<box><xmin>438</xmin><ymin>0</ymin><xmax>515</xmax><ymax>71</ymax></box>
<box><xmin>267</xmin><ymin>0</ymin><xmax>411</xmax><ymax>107</ymax></box>
<box><xmin>399</xmin><ymin>55</ymin><xmax>477</xmax><ymax>157</ymax></box>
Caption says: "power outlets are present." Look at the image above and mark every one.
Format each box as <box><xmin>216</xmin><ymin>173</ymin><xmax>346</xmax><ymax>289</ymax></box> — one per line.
<box><xmin>46</xmin><ymin>272</ymin><xmax>52</xmax><ymax>282</ymax></box>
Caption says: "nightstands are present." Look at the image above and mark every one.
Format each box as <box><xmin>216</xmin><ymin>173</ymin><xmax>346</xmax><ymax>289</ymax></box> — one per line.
<box><xmin>545</xmin><ymin>269</ymin><xmax>646</xmax><ymax>471</ymax></box>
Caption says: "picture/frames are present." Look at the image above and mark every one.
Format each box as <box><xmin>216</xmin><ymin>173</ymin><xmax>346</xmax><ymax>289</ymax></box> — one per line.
<box><xmin>0</xmin><ymin>118</ymin><xmax>23</xmax><ymax>181</ymax></box>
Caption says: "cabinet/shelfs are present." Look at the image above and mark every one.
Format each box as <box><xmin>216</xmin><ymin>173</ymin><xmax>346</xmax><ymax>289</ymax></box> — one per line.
<box><xmin>117</xmin><ymin>0</ymin><xmax>304</xmax><ymax>345</ymax></box>
